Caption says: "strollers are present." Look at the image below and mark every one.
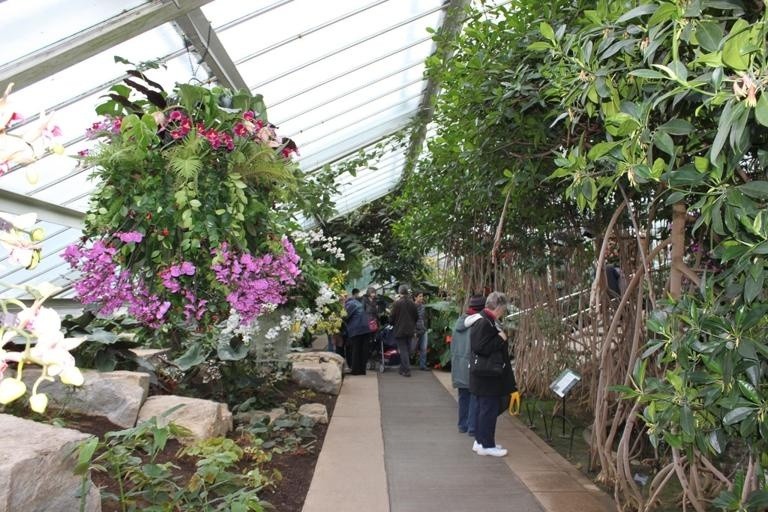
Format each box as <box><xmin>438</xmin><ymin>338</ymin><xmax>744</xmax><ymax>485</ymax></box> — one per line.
<box><xmin>365</xmin><ymin>322</ymin><xmax>403</xmax><ymax>374</ymax></box>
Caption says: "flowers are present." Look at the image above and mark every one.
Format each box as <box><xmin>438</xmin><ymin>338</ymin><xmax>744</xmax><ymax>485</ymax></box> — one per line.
<box><xmin>2</xmin><ymin>58</ymin><xmax>352</xmax><ymax>413</ymax></box>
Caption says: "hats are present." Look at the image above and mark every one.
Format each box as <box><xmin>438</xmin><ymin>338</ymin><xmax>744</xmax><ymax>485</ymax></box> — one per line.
<box><xmin>398</xmin><ymin>285</ymin><xmax>409</xmax><ymax>295</ymax></box>
<box><xmin>366</xmin><ymin>286</ymin><xmax>376</xmax><ymax>296</ymax></box>
<box><xmin>469</xmin><ymin>295</ymin><xmax>485</xmax><ymax>307</ymax></box>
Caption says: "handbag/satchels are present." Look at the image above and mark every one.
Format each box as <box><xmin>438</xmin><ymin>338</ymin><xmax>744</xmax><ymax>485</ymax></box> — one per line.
<box><xmin>471</xmin><ymin>350</ymin><xmax>509</xmax><ymax>377</ymax></box>
<box><xmin>368</xmin><ymin>318</ymin><xmax>379</xmax><ymax>332</ymax></box>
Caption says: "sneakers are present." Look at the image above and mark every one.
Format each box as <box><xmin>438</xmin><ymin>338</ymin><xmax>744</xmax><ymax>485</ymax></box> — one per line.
<box><xmin>468</xmin><ymin>431</ymin><xmax>507</xmax><ymax>457</ymax></box>
<box><xmin>421</xmin><ymin>366</ymin><xmax>430</xmax><ymax>370</ymax></box>
<box><xmin>399</xmin><ymin>369</ymin><xmax>410</xmax><ymax>375</ymax></box>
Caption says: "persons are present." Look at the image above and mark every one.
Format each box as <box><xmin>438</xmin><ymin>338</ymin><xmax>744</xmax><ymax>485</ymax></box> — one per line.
<box><xmin>328</xmin><ymin>285</ymin><xmax>430</xmax><ymax>376</ymax></box>
<box><xmin>464</xmin><ymin>291</ymin><xmax>517</xmax><ymax>457</ymax></box>
<box><xmin>450</xmin><ymin>293</ymin><xmax>486</xmax><ymax>436</ymax></box>
<box><xmin>606</xmin><ymin>266</ymin><xmax>630</xmax><ymax>320</ymax></box>
